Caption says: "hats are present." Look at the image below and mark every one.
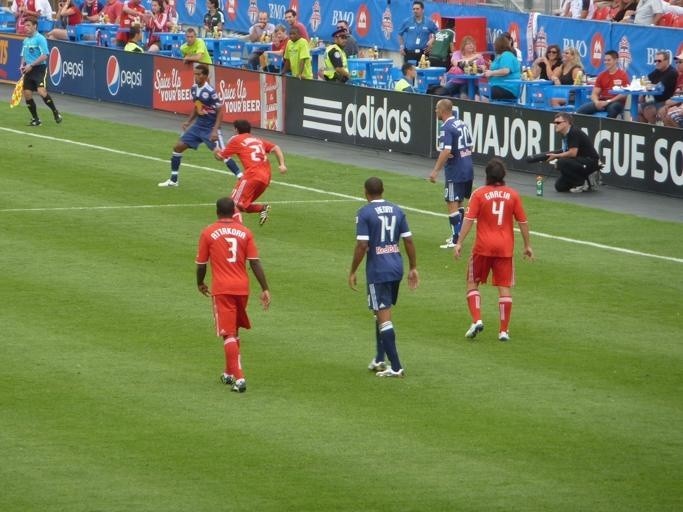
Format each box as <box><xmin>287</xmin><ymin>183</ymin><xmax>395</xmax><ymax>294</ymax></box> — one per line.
<box><xmin>673</xmin><ymin>52</ymin><xmax>683</xmax><ymax>60</ymax></box>
<box><xmin>332</xmin><ymin>29</ymin><xmax>352</xmax><ymax>37</ymax></box>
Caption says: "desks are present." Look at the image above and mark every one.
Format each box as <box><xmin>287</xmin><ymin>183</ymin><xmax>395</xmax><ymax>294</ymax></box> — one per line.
<box><xmin>414</xmin><ymin>66</ymin><xmax>447</xmax><ymax>73</ymax></box>
<box><xmin>448</xmin><ymin>74</ymin><xmax>485</xmax><ymax>100</ymax></box>
<box><xmin>153</xmin><ymin>31</ymin><xmax>186</xmax><ymax>40</ymax></box>
<box><xmin>203</xmin><ymin>37</ymin><xmax>239</xmax><ymax>64</ymax></box>
<box><xmin>80</xmin><ymin>22</ymin><xmax>118</xmax><ymax>30</ymax></box>
<box><xmin>310</xmin><ymin>46</ymin><xmax>326</xmax><ymax>74</ymax></box>
<box><xmin>246</xmin><ymin>41</ymin><xmax>272</xmax><ymax>49</ymax></box>
<box><xmin>504</xmin><ymin>79</ymin><xmax>554</xmax><ymax>108</ymax></box>
<box><xmin>348</xmin><ymin>58</ymin><xmax>393</xmax><ymax>78</ymax></box>
<box><xmin>609</xmin><ymin>90</ymin><xmax>663</xmax><ymax>121</ymax></box>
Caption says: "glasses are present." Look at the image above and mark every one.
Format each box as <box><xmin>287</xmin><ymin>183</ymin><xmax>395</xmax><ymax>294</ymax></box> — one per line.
<box><xmin>654</xmin><ymin>59</ymin><xmax>662</xmax><ymax>63</ymax></box>
<box><xmin>553</xmin><ymin>121</ymin><xmax>565</xmax><ymax>125</ymax></box>
<box><xmin>337</xmin><ymin>26</ymin><xmax>343</xmax><ymax>29</ymax></box>
<box><xmin>547</xmin><ymin>50</ymin><xmax>557</xmax><ymax>54</ymax></box>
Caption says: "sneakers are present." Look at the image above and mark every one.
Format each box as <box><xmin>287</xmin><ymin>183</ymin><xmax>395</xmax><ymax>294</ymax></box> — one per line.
<box><xmin>30</xmin><ymin>119</ymin><xmax>42</xmax><ymax>126</ymax></box>
<box><xmin>497</xmin><ymin>331</ymin><xmax>511</xmax><ymax>340</ymax></box>
<box><xmin>230</xmin><ymin>380</ymin><xmax>247</xmax><ymax>392</ymax></box>
<box><xmin>55</xmin><ymin>113</ymin><xmax>63</xmax><ymax>123</ymax></box>
<box><xmin>464</xmin><ymin>318</ymin><xmax>485</xmax><ymax>339</ymax></box>
<box><xmin>158</xmin><ymin>178</ymin><xmax>179</xmax><ymax>187</ymax></box>
<box><xmin>375</xmin><ymin>366</ymin><xmax>405</xmax><ymax>378</ymax></box>
<box><xmin>569</xmin><ymin>187</ymin><xmax>583</xmax><ymax>193</ymax></box>
<box><xmin>439</xmin><ymin>240</ymin><xmax>457</xmax><ymax>249</ymax></box>
<box><xmin>582</xmin><ymin>178</ymin><xmax>591</xmax><ymax>192</ymax></box>
<box><xmin>368</xmin><ymin>358</ymin><xmax>386</xmax><ymax>372</ymax></box>
<box><xmin>259</xmin><ymin>204</ymin><xmax>270</xmax><ymax>226</ymax></box>
<box><xmin>220</xmin><ymin>374</ymin><xmax>233</xmax><ymax>385</ymax></box>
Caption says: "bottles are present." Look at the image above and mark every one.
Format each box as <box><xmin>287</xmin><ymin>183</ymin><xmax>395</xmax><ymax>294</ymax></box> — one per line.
<box><xmin>464</xmin><ymin>60</ymin><xmax>469</xmax><ymax>74</ymax></box>
<box><xmin>526</xmin><ymin>66</ymin><xmax>533</xmax><ymax>81</ymax></box>
<box><xmin>373</xmin><ymin>45</ymin><xmax>378</xmax><ymax>59</ymax></box>
<box><xmin>472</xmin><ymin>61</ymin><xmax>477</xmax><ymax>73</ymax></box>
<box><xmin>521</xmin><ymin>65</ymin><xmax>526</xmax><ymax>81</ymax></box>
<box><xmin>640</xmin><ymin>75</ymin><xmax>645</xmax><ymax>90</ymax></box>
<box><xmin>536</xmin><ymin>176</ymin><xmax>543</xmax><ymax>196</ymax></box>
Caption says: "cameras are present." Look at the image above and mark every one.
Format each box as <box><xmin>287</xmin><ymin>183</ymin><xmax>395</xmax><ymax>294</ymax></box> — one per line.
<box><xmin>526</xmin><ymin>149</ymin><xmax>563</xmax><ymax>165</ymax></box>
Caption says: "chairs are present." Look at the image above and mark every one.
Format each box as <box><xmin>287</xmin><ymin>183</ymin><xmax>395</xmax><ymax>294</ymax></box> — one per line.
<box><xmin>486</xmin><ymin>80</ymin><xmax>525</xmax><ymax>105</ymax></box>
<box><xmin>543</xmin><ymin>83</ymin><xmax>624</xmax><ymax>118</ymax></box>
<box><xmin>359</xmin><ymin>64</ymin><xmax>391</xmax><ymax>88</ymax></box>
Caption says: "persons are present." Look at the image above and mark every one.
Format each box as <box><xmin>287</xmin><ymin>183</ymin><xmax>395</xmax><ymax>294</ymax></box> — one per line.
<box><xmin>439</xmin><ymin>35</ymin><xmax>486</xmax><ymax>96</ymax></box>
<box><xmin>429</xmin><ymin>22</ymin><xmax>456</xmax><ymax>67</ymax></box>
<box><xmin>214</xmin><ymin>120</ymin><xmax>288</xmax><ymax>226</ymax></box>
<box><xmin>79</xmin><ymin>0</ymin><xmax>104</xmax><ymax>16</ymax></box>
<box><xmin>322</xmin><ymin>29</ymin><xmax>350</xmax><ymax>83</ymax></box>
<box><xmin>124</xmin><ymin>26</ymin><xmax>144</xmax><ymax>52</ymax></box>
<box><xmin>20</xmin><ymin>18</ymin><xmax>63</xmax><ymax>127</ymax></box>
<box><xmin>194</xmin><ymin>197</ymin><xmax>271</xmax><ymax>392</ymax></box>
<box><xmin>247</xmin><ymin>12</ymin><xmax>275</xmax><ymax>69</ymax></box>
<box><xmin>260</xmin><ymin>25</ymin><xmax>289</xmax><ymax>73</ymax></box>
<box><xmin>394</xmin><ymin>64</ymin><xmax>417</xmax><ymax>92</ymax></box>
<box><xmin>455</xmin><ymin>160</ymin><xmax>534</xmax><ymax>340</ymax></box>
<box><xmin>560</xmin><ymin>0</ymin><xmax>683</xmax><ymax>28</ymax></box>
<box><xmin>157</xmin><ymin>65</ymin><xmax>243</xmax><ymax>187</ymax></box>
<box><xmin>47</xmin><ymin>0</ymin><xmax>81</xmax><ymax>42</ymax></box>
<box><xmin>475</xmin><ymin>36</ymin><xmax>521</xmax><ymax>102</ymax></box>
<box><xmin>84</xmin><ymin>0</ymin><xmax>122</xmax><ymax>24</ymax></box>
<box><xmin>532</xmin><ymin>45</ymin><xmax>562</xmax><ymax>80</ymax></box>
<box><xmin>551</xmin><ymin>48</ymin><xmax>582</xmax><ymax>84</ymax></box>
<box><xmin>638</xmin><ymin>52</ymin><xmax>677</xmax><ymax>123</ymax></box>
<box><xmin>337</xmin><ymin>20</ymin><xmax>359</xmax><ymax>56</ymax></box>
<box><xmin>280</xmin><ymin>26</ymin><xmax>314</xmax><ymax>80</ymax></box>
<box><xmin>271</xmin><ymin>36</ymin><xmax>280</xmax><ymax>51</ymax></box>
<box><xmin>11</xmin><ymin>0</ymin><xmax>41</xmax><ymax>35</ymax></box>
<box><xmin>348</xmin><ymin>177</ymin><xmax>420</xmax><ymax>378</ymax></box>
<box><xmin>116</xmin><ymin>0</ymin><xmax>146</xmax><ymax>47</ymax></box>
<box><xmin>430</xmin><ymin>98</ymin><xmax>473</xmax><ymax>248</ymax></box>
<box><xmin>284</xmin><ymin>9</ymin><xmax>310</xmax><ymax>41</ymax></box>
<box><xmin>35</xmin><ymin>0</ymin><xmax>57</xmax><ymax>33</ymax></box>
<box><xmin>144</xmin><ymin>0</ymin><xmax>168</xmax><ymax>51</ymax></box>
<box><xmin>398</xmin><ymin>2</ymin><xmax>439</xmax><ymax>66</ymax></box>
<box><xmin>203</xmin><ymin>0</ymin><xmax>225</xmax><ymax>32</ymax></box>
<box><xmin>576</xmin><ymin>50</ymin><xmax>629</xmax><ymax>118</ymax></box>
<box><xmin>179</xmin><ymin>28</ymin><xmax>212</xmax><ymax>64</ymax></box>
<box><xmin>657</xmin><ymin>52</ymin><xmax>683</xmax><ymax>127</ymax></box>
<box><xmin>545</xmin><ymin>113</ymin><xmax>598</xmax><ymax>194</ymax></box>
